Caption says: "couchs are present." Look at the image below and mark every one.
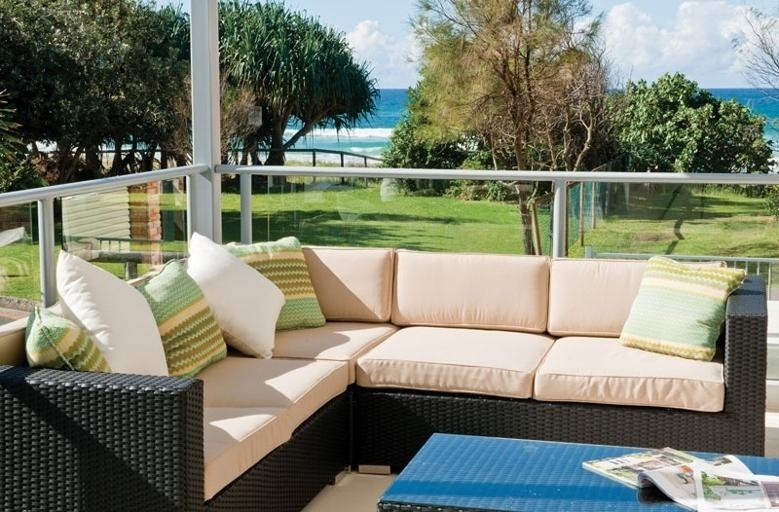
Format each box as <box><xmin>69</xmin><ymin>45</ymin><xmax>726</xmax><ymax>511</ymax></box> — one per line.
<box><xmin>1</xmin><ymin>273</ymin><xmax>347</xmax><ymax>512</ymax></box>
<box><xmin>224</xmin><ymin>245</ymin><xmax>767</xmax><ymax>474</ymax></box>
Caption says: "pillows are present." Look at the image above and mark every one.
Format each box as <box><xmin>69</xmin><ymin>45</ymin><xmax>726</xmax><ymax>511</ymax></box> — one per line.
<box><xmin>548</xmin><ymin>255</ymin><xmax>727</xmax><ymax>339</ymax></box>
<box><xmin>619</xmin><ymin>255</ymin><xmax>746</xmax><ymax>362</ymax></box>
<box><xmin>25</xmin><ymin>304</ymin><xmax>112</xmax><ymax>373</ymax></box>
<box><xmin>56</xmin><ymin>250</ymin><xmax>168</xmax><ymax>376</ymax></box>
<box><xmin>389</xmin><ymin>247</ymin><xmax>551</xmax><ymax>335</ymax></box>
<box><xmin>302</xmin><ymin>244</ymin><xmax>395</xmax><ymax>323</ymax></box>
<box><xmin>228</xmin><ymin>236</ymin><xmax>325</xmax><ymax>331</ymax></box>
<box><xmin>186</xmin><ymin>232</ymin><xmax>286</xmax><ymax>359</ymax></box>
<box><xmin>135</xmin><ymin>258</ymin><xmax>227</xmax><ymax>378</ymax></box>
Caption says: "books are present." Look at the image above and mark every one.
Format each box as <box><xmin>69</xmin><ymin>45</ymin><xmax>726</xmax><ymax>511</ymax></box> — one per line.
<box><xmin>581</xmin><ymin>446</ymin><xmax>757</xmax><ymax>512</ymax></box>
